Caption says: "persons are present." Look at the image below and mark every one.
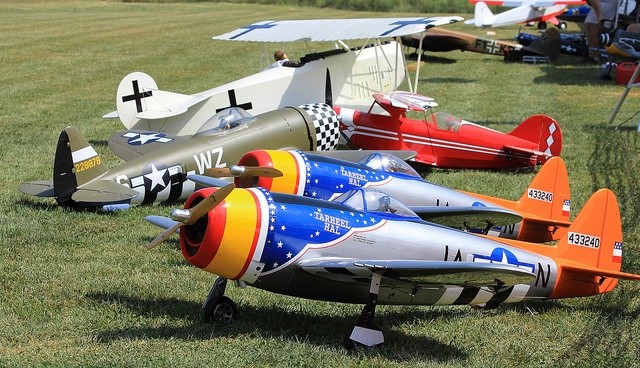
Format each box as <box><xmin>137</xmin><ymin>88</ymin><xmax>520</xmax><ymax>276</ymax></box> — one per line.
<box><xmin>585</xmin><ymin>0</ymin><xmax>621</xmax><ymax>64</ymax></box>
<box><xmin>268</xmin><ymin>50</ymin><xmax>289</xmax><ymax>68</ymax></box>
<box><xmin>627</xmin><ymin>0</ymin><xmax>640</xmax><ymax>34</ymax></box>
